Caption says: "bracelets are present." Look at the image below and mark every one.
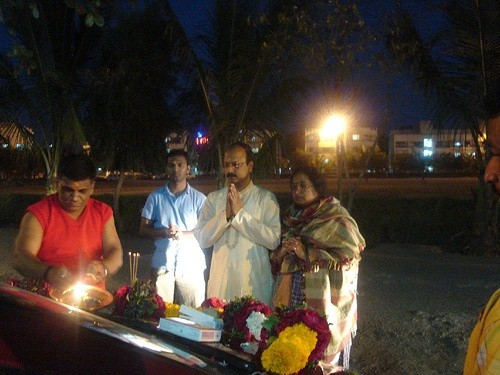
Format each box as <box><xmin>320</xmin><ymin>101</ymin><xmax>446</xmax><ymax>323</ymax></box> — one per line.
<box><xmin>43</xmin><ymin>266</ymin><xmax>53</xmax><ymax>280</ymax></box>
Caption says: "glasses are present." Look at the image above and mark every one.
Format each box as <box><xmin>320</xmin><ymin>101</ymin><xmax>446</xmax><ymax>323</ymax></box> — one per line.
<box><xmin>289</xmin><ymin>183</ymin><xmax>316</xmax><ymax>192</ymax></box>
<box><xmin>224</xmin><ymin>161</ymin><xmax>248</xmax><ymax>169</ymax></box>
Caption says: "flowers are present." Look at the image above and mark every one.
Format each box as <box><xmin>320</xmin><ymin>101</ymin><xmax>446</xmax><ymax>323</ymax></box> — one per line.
<box><xmin>196</xmin><ymin>296</ymin><xmax>333</xmax><ymax>375</ymax></box>
<box><xmin>112</xmin><ymin>277</ymin><xmax>166</xmax><ymax>320</ymax></box>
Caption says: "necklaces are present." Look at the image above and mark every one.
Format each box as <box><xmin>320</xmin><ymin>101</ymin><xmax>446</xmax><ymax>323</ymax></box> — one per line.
<box><xmin>225</xmin><ymin>227</ymin><xmax>238</xmax><ymax>249</ymax></box>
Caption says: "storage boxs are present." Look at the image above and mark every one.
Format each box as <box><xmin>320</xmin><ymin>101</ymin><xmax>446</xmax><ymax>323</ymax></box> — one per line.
<box><xmin>159</xmin><ymin>304</ymin><xmax>225</xmax><ymax>343</ymax></box>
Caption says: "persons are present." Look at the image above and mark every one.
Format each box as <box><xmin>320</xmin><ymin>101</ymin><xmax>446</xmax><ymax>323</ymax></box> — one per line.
<box><xmin>270</xmin><ymin>165</ymin><xmax>366</xmax><ymax>375</ymax></box>
<box><xmin>462</xmin><ymin>85</ymin><xmax>500</xmax><ymax>375</ymax></box>
<box><xmin>12</xmin><ymin>153</ymin><xmax>123</xmax><ymax>290</ymax></box>
<box><xmin>141</xmin><ymin>148</ymin><xmax>207</xmax><ymax>305</ymax></box>
<box><xmin>194</xmin><ymin>142</ymin><xmax>281</xmax><ymax>308</ymax></box>
